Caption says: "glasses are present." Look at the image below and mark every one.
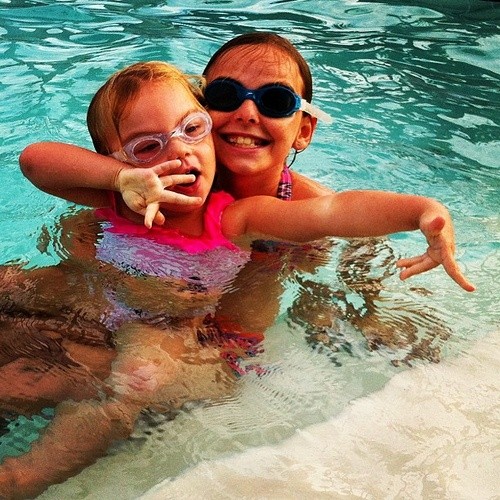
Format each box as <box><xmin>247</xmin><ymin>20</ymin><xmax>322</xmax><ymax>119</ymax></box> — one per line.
<box><xmin>106</xmin><ymin>112</ymin><xmax>213</xmax><ymax>170</ymax></box>
<box><xmin>200</xmin><ymin>75</ymin><xmax>333</xmax><ymax>124</ymax></box>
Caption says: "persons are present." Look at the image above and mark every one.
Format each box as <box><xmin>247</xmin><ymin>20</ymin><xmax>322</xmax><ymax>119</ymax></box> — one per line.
<box><xmin>0</xmin><ymin>33</ymin><xmax>335</xmax><ymax>409</ymax></box>
<box><xmin>0</xmin><ymin>60</ymin><xmax>476</xmax><ymax>500</ymax></box>
